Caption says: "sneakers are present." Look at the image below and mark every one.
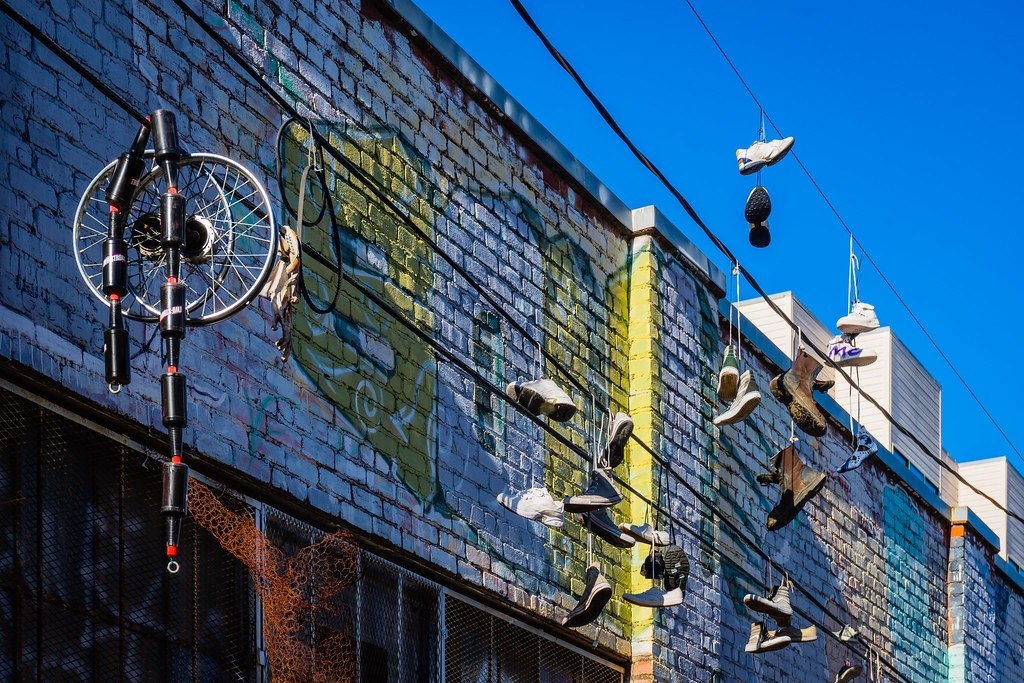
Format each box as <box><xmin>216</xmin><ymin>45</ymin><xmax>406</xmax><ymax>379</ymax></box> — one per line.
<box><xmin>496</xmin><ymin>487</ymin><xmax>566</xmax><ymax>528</ymax></box>
<box><xmin>505</xmin><ymin>375</ymin><xmax>576</xmax><ymax>422</ymax></box>
<box><xmin>823</xmin><ymin>335</ymin><xmax>878</xmax><ymax>366</ymax></box>
<box><xmin>836</xmin><ymin>302</ymin><xmax>880</xmax><ymax>333</ymax></box>
<box><xmin>736</xmin><ymin>136</ymin><xmax>794</xmax><ymax>174</ymax></box>
<box><xmin>561</xmin><ymin>412</ymin><xmax>690</xmax><ymax>627</ymax></box>
<box><xmin>832</xmin><ymin>623</ymin><xmax>859</xmax><ymax>643</ymax></box>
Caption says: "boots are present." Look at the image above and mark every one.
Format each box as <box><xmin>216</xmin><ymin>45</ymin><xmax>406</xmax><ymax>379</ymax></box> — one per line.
<box><xmin>745</xmin><ymin>621</ymin><xmax>791</xmax><ymax>653</ymax></box>
<box><xmin>713</xmin><ymin>369</ymin><xmax>761</xmax><ymax>425</ymax></box>
<box><xmin>717</xmin><ymin>345</ymin><xmax>740</xmax><ymax>401</ymax></box>
<box><xmin>769</xmin><ymin>347</ymin><xmax>835</xmax><ymax>437</ymax></box>
<box><xmin>755</xmin><ymin>440</ymin><xmax>826</xmax><ymax>531</ymax></box>
<box><xmin>743</xmin><ymin>585</ymin><xmax>793</xmax><ymax>617</ymax></box>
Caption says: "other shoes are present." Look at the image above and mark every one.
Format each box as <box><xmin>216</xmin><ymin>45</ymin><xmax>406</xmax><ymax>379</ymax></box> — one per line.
<box><xmin>836</xmin><ymin>425</ymin><xmax>879</xmax><ymax>473</ymax></box>
<box><xmin>833</xmin><ymin>664</ymin><xmax>863</xmax><ymax>683</ymax></box>
<box><xmin>765</xmin><ymin>623</ymin><xmax>817</xmax><ymax>642</ymax></box>
<box><xmin>744</xmin><ymin>186</ymin><xmax>772</xmax><ymax>248</ymax></box>
